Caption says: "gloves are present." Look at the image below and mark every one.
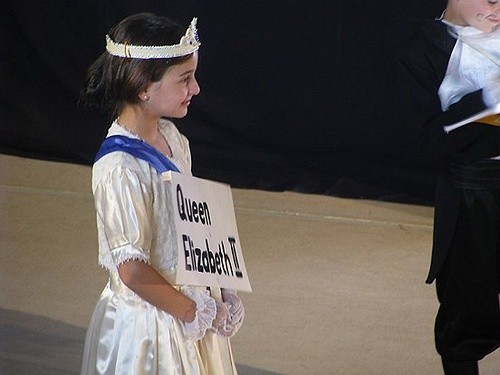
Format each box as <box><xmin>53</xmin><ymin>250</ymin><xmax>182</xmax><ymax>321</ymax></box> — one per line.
<box><xmin>220</xmin><ymin>287</ymin><xmax>246</xmax><ymax>330</ymax></box>
<box><xmin>183</xmin><ymin>289</ymin><xmax>235</xmax><ymax>343</ymax></box>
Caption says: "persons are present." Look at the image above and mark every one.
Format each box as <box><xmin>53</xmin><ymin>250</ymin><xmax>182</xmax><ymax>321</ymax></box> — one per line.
<box><xmin>424</xmin><ymin>1</ymin><xmax>500</xmax><ymax>375</ymax></box>
<box><xmin>76</xmin><ymin>7</ymin><xmax>247</xmax><ymax>375</ymax></box>
